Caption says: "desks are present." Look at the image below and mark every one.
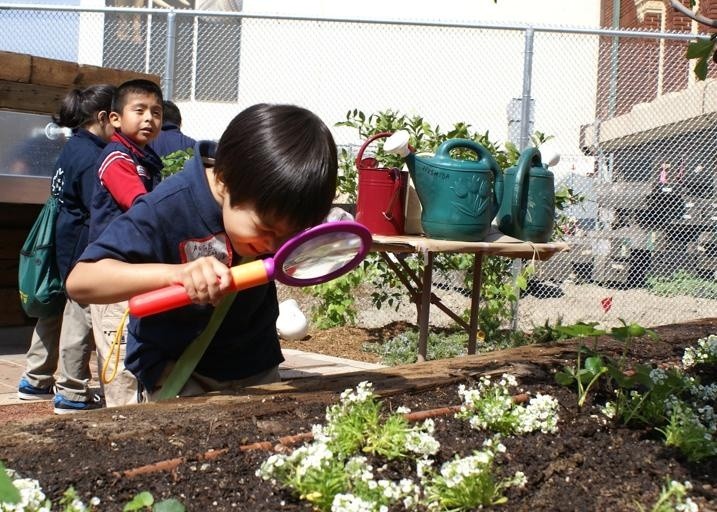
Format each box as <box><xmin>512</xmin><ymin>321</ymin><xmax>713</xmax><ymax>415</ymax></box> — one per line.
<box><xmin>369</xmin><ymin>234</ymin><xmax>561</xmax><ymax>364</ymax></box>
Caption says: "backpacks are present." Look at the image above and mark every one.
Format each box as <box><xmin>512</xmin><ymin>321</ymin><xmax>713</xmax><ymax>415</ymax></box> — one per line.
<box><xmin>18</xmin><ymin>197</ymin><xmax>66</xmax><ymax>318</ymax></box>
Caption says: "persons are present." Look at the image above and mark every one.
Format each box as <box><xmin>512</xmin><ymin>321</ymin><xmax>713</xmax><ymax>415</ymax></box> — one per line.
<box><xmin>63</xmin><ymin>102</ymin><xmax>339</xmax><ymax>405</ymax></box>
<box><xmin>9</xmin><ymin>116</ymin><xmax>65</xmax><ymax>177</ymax></box>
<box><xmin>144</xmin><ymin>100</ymin><xmax>201</xmax><ymax>159</ymax></box>
<box><xmin>84</xmin><ymin>79</ymin><xmax>166</xmax><ymax>410</ymax></box>
<box><xmin>19</xmin><ymin>82</ymin><xmax>118</xmax><ymax>417</ymax></box>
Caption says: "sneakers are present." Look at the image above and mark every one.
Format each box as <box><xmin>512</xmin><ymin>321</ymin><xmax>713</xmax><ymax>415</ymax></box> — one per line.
<box><xmin>54</xmin><ymin>393</ymin><xmax>103</xmax><ymax>415</ymax></box>
<box><xmin>19</xmin><ymin>379</ymin><xmax>56</xmax><ymax>400</ymax></box>
